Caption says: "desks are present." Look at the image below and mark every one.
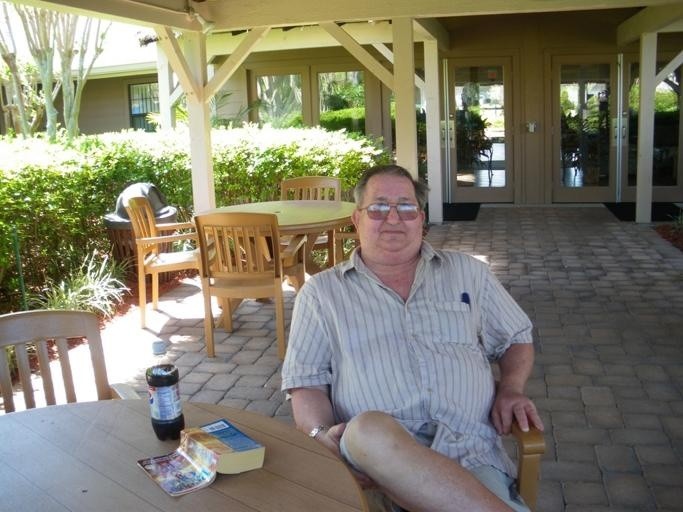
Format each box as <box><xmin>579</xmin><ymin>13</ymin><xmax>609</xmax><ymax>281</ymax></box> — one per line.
<box><xmin>0</xmin><ymin>399</ymin><xmax>369</xmax><ymax>512</ymax></box>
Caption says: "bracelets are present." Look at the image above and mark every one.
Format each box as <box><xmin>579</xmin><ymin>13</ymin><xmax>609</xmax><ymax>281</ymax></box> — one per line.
<box><xmin>309</xmin><ymin>425</ymin><xmax>331</xmax><ymax>438</ymax></box>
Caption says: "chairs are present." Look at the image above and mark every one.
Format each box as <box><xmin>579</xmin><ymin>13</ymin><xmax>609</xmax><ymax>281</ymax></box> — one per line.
<box><xmin>0</xmin><ymin>309</ymin><xmax>142</xmax><ymax>413</ymax></box>
<box><xmin>358</xmin><ymin>380</ymin><xmax>545</xmax><ymax>512</ymax></box>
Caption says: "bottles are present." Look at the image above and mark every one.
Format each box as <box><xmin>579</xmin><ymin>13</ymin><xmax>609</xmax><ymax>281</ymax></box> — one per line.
<box><xmin>145</xmin><ymin>340</ymin><xmax>185</xmax><ymax>442</ymax></box>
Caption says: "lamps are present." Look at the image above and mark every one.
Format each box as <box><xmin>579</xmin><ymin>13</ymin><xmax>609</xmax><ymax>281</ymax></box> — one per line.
<box><xmin>186</xmin><ymin>7</ymin><xmax>216</xmax><ymax>36</ymax></box>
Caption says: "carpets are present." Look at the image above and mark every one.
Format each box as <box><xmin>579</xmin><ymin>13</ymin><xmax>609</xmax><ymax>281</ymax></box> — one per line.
<box><xmin>424</xmin><ymin>203</ymin><xmax>480</xmax><ymax>221</ymax></box>
<box><xmin>603</xmin><ymin>203</ymin><xmax>683</xmax><ymax>222</ymax></box>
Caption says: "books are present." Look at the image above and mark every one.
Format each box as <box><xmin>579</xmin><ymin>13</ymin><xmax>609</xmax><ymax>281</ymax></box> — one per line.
<box><xmin>136</xmin><ymin>419</ymin><xmax>266</xmax><ymax>497</ymax></box>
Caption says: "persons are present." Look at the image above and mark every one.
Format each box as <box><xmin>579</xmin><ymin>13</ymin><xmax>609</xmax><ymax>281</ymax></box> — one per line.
<box><xmin>281</xmin><ymin>164</ymin><xmax>545</xmax><ymax>512</ymax></box>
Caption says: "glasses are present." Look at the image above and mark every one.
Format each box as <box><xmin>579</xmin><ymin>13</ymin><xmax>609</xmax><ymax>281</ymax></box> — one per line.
<box><xmin>358</xmin><ymin>203</ymin><xmax>420</xmax><ymax>221</ymax></box>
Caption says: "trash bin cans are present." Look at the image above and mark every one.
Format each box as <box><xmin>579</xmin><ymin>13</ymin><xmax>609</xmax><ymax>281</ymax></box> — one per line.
<box><xmin>102</xmin><ymin>182</ymin><xmax>177</xmax><ymax>299</ymax></box>
